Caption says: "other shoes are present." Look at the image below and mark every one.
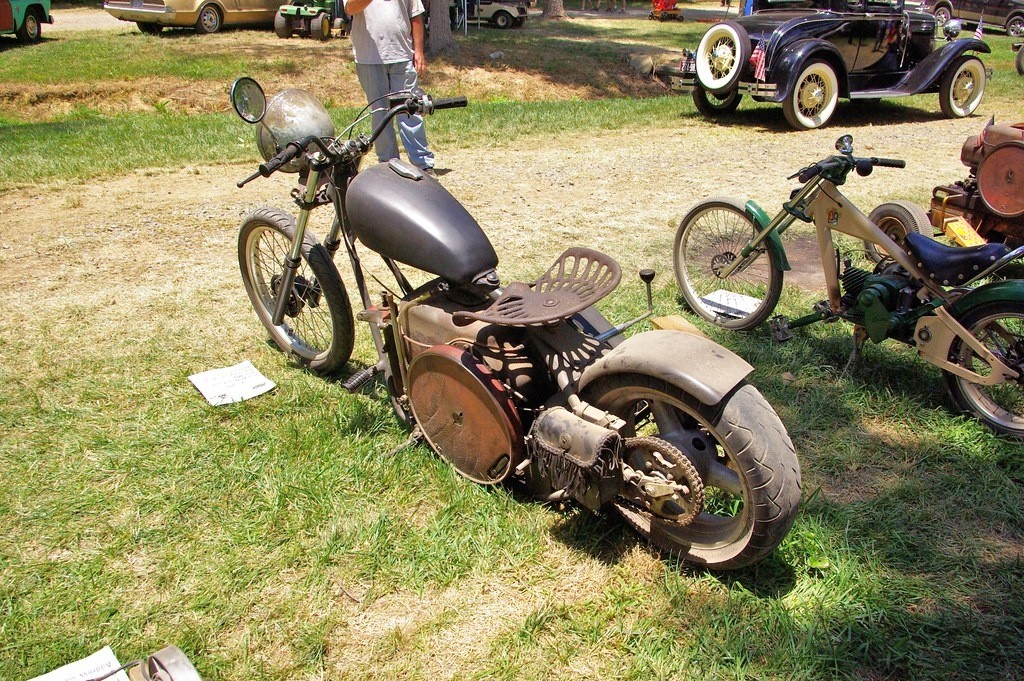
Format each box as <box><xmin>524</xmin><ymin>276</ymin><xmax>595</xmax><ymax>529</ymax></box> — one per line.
<box><xmin>423</xmin><ymin>167</ymin><xmax>439</xmax><ymax>181</ymax></box>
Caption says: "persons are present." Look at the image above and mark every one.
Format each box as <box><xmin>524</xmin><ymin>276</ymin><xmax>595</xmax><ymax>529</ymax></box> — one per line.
<box><xmin>720</xmin><ymin>0</ymin><xmax>731</xmax><ymax>7</ymax></box>
<box><xmin>582</xmin><ymin>0</ymin><xmax>626</xmax><ymax>14</ymax></box>
<box><xmin>344</xmin><ymin>0</ymin><xmax>437</xmax><ymax>180</ymax></box>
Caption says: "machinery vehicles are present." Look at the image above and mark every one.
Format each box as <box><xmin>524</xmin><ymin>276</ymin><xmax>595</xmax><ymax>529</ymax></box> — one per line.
<box><xmin>273</xmin><ymin>0</ymin><xmax>349</xmax><ymax>42</ymax></box>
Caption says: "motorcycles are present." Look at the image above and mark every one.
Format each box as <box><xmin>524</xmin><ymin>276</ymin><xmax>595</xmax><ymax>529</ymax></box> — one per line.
<box><xmin>228</xmin><ymin>74</ymin><xmax>803</xmax><ymax>572</ymax></box>
<box><xmin>672</xmin><ymin>132</ymin><xmax>1024</xmax><ymax>446</ymax></box>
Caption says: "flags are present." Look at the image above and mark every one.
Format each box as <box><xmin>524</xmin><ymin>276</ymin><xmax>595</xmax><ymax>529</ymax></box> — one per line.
<box><xmin>750</xmin><ymin>34</ymin><xmax>766</xmax><ymax>82</ymax></box>
<box><xmin>972</xmin><ymin>15</ymin><xmax>983</xmax><ymax>54</ymax></box>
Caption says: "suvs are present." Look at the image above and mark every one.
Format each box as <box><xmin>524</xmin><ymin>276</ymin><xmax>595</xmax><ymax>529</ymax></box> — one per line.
<box><xmin>448</xmin><ymin>0</ymin><xmax>529</xmax><ymax>29</ymax></box>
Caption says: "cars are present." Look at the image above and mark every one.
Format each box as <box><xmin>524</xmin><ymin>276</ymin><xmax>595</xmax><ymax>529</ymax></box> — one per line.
<box><xmin>657</xmin><ymin>0</ymin><xmax>993</xmax><ymax>133</ymax></box>
<box><xmin>923</xmin><ymin>0</ymin><xmax>1024</xmax><ymax>39</ymax></box>
<box><xmin>103</xmin><ymin>0</ymin><xmax>292</xmax><ymax>35</ymax></box>
<box><xmin>0</xmin><ymin>0</ymin><xmax>54</xmax><ymax>45</ymax></box>
<box><xmin>927</xmin><ymin>113</ymin><xmax>1024</xmax><ymax>249</ymax></box>
